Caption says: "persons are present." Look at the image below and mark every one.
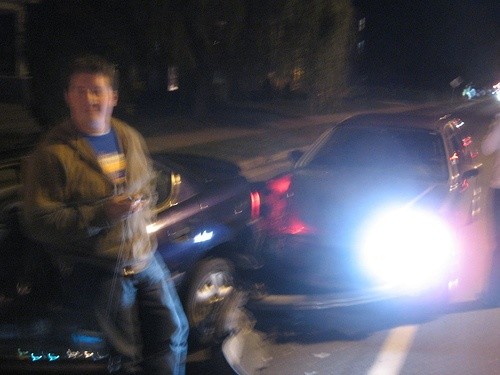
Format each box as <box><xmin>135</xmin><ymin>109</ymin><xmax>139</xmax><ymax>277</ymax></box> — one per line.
<box><xmin>16</xmin><ymin>54</ymin><xmax>190</xmax><ymax>374</ymax></box>
<box><xmin>473</xmin><ymin>112</ymin><xmax>500</xmax><ymax>309</ymax></box>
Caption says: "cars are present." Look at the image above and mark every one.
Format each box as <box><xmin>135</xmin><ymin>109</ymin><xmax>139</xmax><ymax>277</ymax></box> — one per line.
<box><xmin>2</xmin><ymin>143</ymin><xmax>263</xmax><ymax>358</ymax></box>
<box><xmin>257</xmin><ymin>106</ymin><xmax>490</xmax><ymax>325</ymax></box>
